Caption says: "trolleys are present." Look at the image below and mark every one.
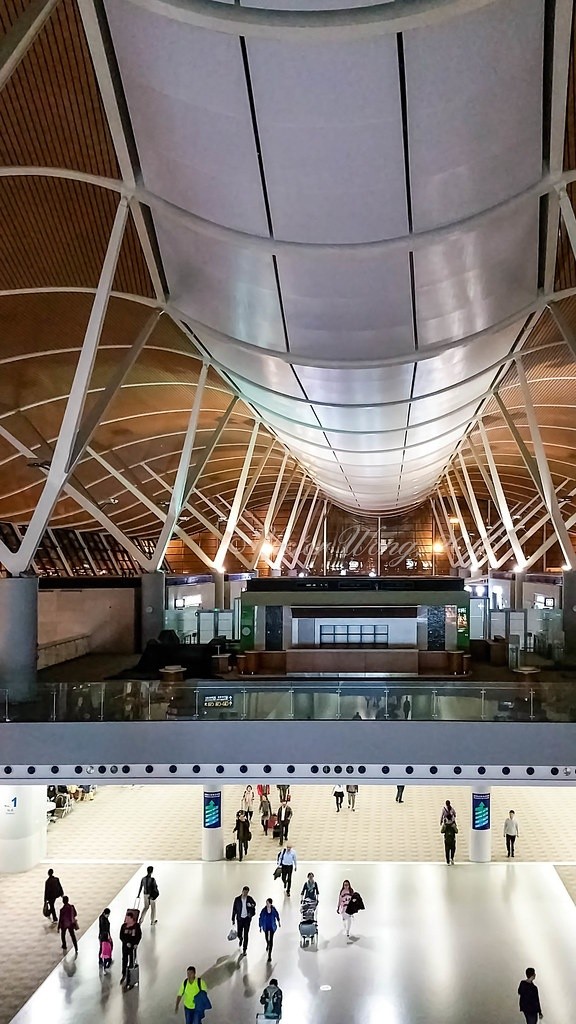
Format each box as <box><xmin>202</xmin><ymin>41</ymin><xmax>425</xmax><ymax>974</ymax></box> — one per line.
<box><xmin>256</xmin><ymin>1013</ymin><xmax>279</xmax><ymax>1024</ymax></box>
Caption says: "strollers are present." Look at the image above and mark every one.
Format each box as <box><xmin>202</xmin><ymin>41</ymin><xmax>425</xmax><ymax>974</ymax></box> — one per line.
<box><xmin>299</xmin><ymin>900</ymin><xmax>318</xmax><ymax>948</ymax></box>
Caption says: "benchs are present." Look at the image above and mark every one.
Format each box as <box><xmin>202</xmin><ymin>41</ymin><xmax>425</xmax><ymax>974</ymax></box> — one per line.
<box><xmin>54</xmin><ymin>793</ymin><xmax>74</xmax><ymax>818</ymax></box>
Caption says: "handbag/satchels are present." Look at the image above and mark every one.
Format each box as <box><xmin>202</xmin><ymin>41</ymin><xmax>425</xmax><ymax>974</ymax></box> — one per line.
<box><xmin>73</xmin><ymin>918</ymin><xmax>79</xmax><ymax>930</ymax></box>
<box><xmin>43</xmin><ymin>901</ymin><xmax>51</xmax><ymax>917</ymax></box>
<box><xmin>249</xmin><ymin>832</ymin><xmax>252</xmax><ymax>841</ymax></box>
<box><xmin>277</xmin><ymin>848</ymin><xmax>286</xmax><ymax>865</ymax></box>
<box><xmin>227</xmin><ymin>925</ymin><xmax>237</xmax><ymax>941</ymax></box>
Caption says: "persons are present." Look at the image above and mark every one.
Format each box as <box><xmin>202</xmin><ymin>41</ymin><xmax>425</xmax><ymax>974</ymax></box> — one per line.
<box><xmin>331</xmin><ymin>784</ymin><xmax>344</xmax><ymax>812</ymax></box>
<box><xmin>395</xmin><ymin>784</ymin><xmax>405</xmax><ymax>803</ymax></box>
<box><xmin>137</xmin><ymin>866</ymin><xmax>157</xmax><ymax>925</ymax></box>
<box><xmin>120</xmin><ymin>913</ymin><xmax>142</xmax><ymax>990</ymax></box>
<box><xmin>504</xmin><ymin>810</ymin><xmax>519</xmax><ymax>857</ymax></box>
<box><xmin>56</xmin><ymin>896</ymin><xmax>78</xmax><ymax>954</ymax></box>
<box><xmin>233</xmin><ymin>784</ymin><xmax>293</xmax><ymax>862</ymax></box>
<box><xmin>99</xmin><ymin>908</ymin><xmax>112</xmax><ymax>969</ymax></box>
<box><xmin>352</xmin><ymin>712</ymin><xmax>362</xmax><ymax>720</ymax></box>
<box><xmin>278</xmin><ymin>843</ymin><xmax>297</xmax><ymax>896</ymax></box>
<box><xmin>47</xmin><ymin>785</ymin><xmax>94</xmax><ymax>821</ymax></box>
<box><xmin>44</xmin><ymin>869</ymin><xmax>64</xmax><ymax>923</ymax></box>
<box><xmin>260</xmin><ymin>979</ymin><xmax>282</xmax><ymax>1018</ymax></box>
<box><xmin>441</xmin><ymin>815</ymin><xmax>458</xmax><ymax>865</ymax></box>
<box><xmin>175</xmin><ymin>966</ymin><xmax>208</xmax><ymax>1024</ymax></box>
<box><xmin>301</xmin><ymin>873</ymin><xmax>319</xmax><ymax>919</ymax></box>
<box><xmin>366</xmin><ymin>696</ymin><xmax>411</xmax><ymax>719</ymax></box>
<box><xmin>259</xmin><ymin>898</ymin><xmax>281</xmax><ymax>962</ymax></box>
<box><xmin>346</xmin><ymin>785</ymin><xmax>359</xmax><ymax>812</ymax></box>
<box><xmin>232</xmin><ymin>886</ymin><xmax>256</xmax><ymax>956</ymax></box>
<box><xmin>440</xmin><ymin>800</ymin><xmax>457</xmax><ymax>825</ymax></box>
<box><xmin>337</xmin><ymin>880</ymin><xmax>357</xmax><ymax>943</ymax></box>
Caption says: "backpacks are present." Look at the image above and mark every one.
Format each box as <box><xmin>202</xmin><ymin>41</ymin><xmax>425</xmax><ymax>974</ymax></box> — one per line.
<box><xmin>148</xmin><ymin>878</ymin><xmax>159</xmax><ymax>905</ymax></box>
<box><xmin>444</xmin><ymin>823</ymin><xmax>455</xmax><ymax>840</ymax></box>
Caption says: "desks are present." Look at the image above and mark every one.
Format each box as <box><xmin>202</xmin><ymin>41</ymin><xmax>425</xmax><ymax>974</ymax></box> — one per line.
<box><xmin>212</xmin><ymin>654</ymin><xmax>233</xmax><ymax>674</ymax></box>
<box><xmin>47</xmin><ymin>801</ymin><xmax>57</xmax><ymax>825</ymax></box>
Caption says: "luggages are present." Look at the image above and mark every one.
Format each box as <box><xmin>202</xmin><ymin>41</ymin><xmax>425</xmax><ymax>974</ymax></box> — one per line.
<box><xmin>124</xmin><ymin>897</ymin><xmax>141</xmax><ymax>923</ymax></box>
<box><xmin>268</xmin><ymin>813</ymin><xmax>281</xmax><ymax>839</ymax></box>
<box><xmin>226</xmin><ymin>831</ymin><xmax>236</xmax><ymax>860</ymax></box>
<box><xmin>126</xmin><ymin>948</ymin><xmax>139</xmax><ymax>990</ymax></box>
<box><xmin>287</xmin><ymin>788</ymin><xmax>291</xmax><ymax>802</ymax></box>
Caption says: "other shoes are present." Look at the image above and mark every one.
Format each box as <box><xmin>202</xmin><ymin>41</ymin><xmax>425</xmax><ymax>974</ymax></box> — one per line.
<box><xmin>103</xmin><ymin>968</ymin><xmax>110</xmax><ymax>974</ymax></box>
<box><xmin>284</xmin><ymin>880</ymin><xmax>287</xmax><ymax>888</ymax></box>
<box><xmin>62</xmin><ymin>945</ymin><xmax>67</xmax><ymax>950</ymax></box>
<box><xmin>75</xmin><ymin>947</ymin><xmax>78</xmax><ymax>951</ymax></box>
<box><xmin>243</xmin><ymin>949</ymin><xmax>247</xmax><ymax>956</ymax></box>
<box><xmin>239</xmin><ymin>937</ymin><xmax>243</xmax><ymax>946</ymax></box>
<box><xmin>120</xmin><ymin>973</ymin><xmax>124</xmax><ymax>983</ymax></box>
<box><xmin>151</xmin><ymin>920</ymin><xmax>158</xmax><ymax>925</ymax></box>
<box><xmin>47</xmin><ymin>916</ymin><xmax>50</xmax><ymax>919</ymax></box>
<box><xmin>287</xmin><ymin>890</ymin><xmax>290</xmax><ymax>897</ymax></box>
<box><xmin>51</xmin><ymin>920</ymin><xmax>58</xmax><ymax>924</ymax></box>
<box><xmin>268</xmin><ymin>954</ymin><xmax>272</xmax><ymax>962</ymax></box>
<box><xmin>266</xmin><ymin>944</ymin><xmax>268</xmax><ymax>951</ymax></box>
<box><xmin>99</xmin><ymin>961</ymin><xmax>104</xmax><ymax>967</ymax></box>
